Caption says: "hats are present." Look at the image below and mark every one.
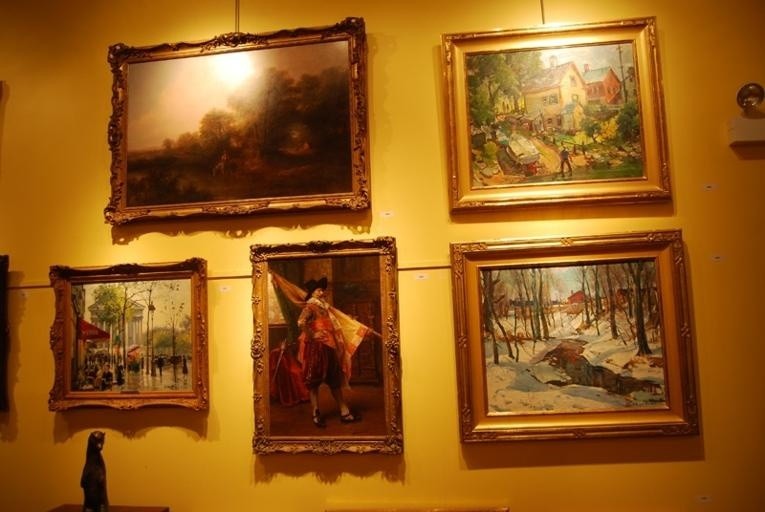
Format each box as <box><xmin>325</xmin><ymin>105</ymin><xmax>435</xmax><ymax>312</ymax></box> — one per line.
<box><xmin>303</xmin><ymin>277</ymin><xmax>327</xmax><ymax>302</ymax></box>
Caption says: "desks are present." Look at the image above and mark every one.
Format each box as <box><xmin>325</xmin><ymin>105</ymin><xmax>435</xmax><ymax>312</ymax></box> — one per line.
<box><xmin>46</xmin><ymin>504</ymin><xmax>169</xmax><ymax>512</ymax></box>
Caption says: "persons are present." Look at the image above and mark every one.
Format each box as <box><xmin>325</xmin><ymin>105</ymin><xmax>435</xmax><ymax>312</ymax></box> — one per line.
<box><xmin>78</xmin><ymin>350</ymin><xmax>188</xmax><ymax>392</ymax></box>
<box><xmin>293</xmin><ymin>276</ymin><xmax>357</xmax><ymax>426</ymax></box>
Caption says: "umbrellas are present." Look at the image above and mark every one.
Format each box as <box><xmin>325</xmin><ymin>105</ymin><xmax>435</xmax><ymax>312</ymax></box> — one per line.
<box><xmin>79</xmin><ymin>320</ymin><xmax>110</xmax><ymax>342</ymax></box>
<box><xmin>128</xmin><ymin>344</ymin><xmax>139</xmax><ymax>354</ymax></box>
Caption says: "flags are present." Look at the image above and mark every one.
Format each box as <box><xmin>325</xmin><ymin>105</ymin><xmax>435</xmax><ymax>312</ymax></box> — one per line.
<box><xmin>267</xmin><ymin>272</ymin><xmax>369</xmax><ymax>392</ymax></box>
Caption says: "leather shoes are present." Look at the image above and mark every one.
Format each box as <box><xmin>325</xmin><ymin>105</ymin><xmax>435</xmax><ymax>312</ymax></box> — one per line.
<box><xmin>312</xmin><ymin>409</ymin><xmax>321</xmax><ymax>427</ymax></box>
<box><xmin>340</xmin><ymin>416</ymin><xmax>361</xmax><ymax>423</ymax></box>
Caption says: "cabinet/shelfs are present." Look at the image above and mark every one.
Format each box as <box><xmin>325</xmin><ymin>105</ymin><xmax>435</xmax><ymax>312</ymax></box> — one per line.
<box><xmin>335</xmin><ymin>299</ymin><xmax>380</xmax><ymax>384</ymax></box>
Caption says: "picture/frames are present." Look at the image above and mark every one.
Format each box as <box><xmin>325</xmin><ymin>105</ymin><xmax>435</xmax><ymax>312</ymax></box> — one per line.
<box><xmin>48</xmin><ymin>257</ymin><xmax>209</xmax><ymax>413</ymax></box>
<box><xmin>442</xmin><ymin>16</ymin><xmax>672</xmax><ymax>214</ymax></box>
<box><xmin>448</xmin><ymin>230</ymin><xmax>701</xmax><ymax>444</ymax></box>
<box><xmin>0</xmin><ymin>255</ymin><xmax>9</xmax><ymax>411</ymax></box>
<box><xmin>249</xmin><ymin>237</ymin><xmax>404</xmax><ymax>456</ymax></box>
<box><xmin>103</xmin><ymin>16</ymin><xmax>371</xmax><ymax>225</ymax></box>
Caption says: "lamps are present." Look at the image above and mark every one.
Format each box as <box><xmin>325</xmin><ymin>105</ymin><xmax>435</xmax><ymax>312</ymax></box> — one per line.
<box><xmin>727</xmin><ymin>82</ymin><xmax>765</xmax><ymax>146</ymax></box>
